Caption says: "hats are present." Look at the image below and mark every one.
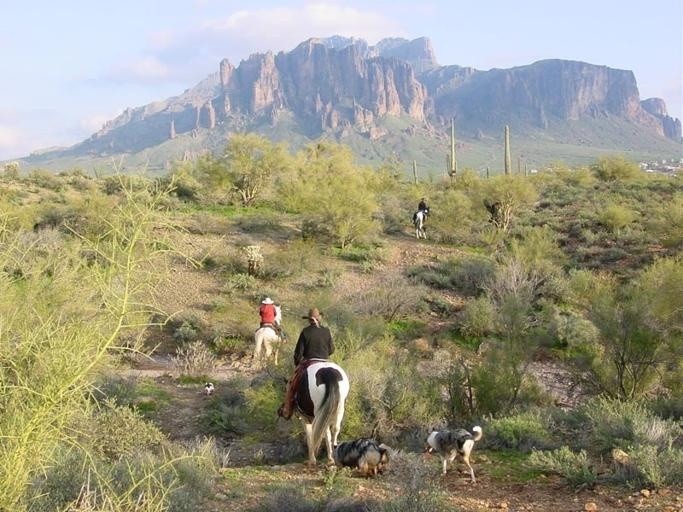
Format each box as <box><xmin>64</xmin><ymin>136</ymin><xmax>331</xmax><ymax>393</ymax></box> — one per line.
<box><xmin>301</xmin><ymin>308</ymin><xmax>323</xmax><ymax>322</ymax></box>
<box><xmin>260</xmin><ymin>297</ymin><xmax>274</xmax><ymax>306</ymax></box>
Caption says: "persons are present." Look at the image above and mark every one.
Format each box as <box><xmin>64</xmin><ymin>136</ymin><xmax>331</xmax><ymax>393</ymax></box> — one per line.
<box><xmin>419</xmin><ymin>198</ymin><xmax>428</xmax><ymax>221</ymax></box>
<box><xmin>279</xmin><ymin>308</ymin><xmax>335</xmax><ymax>420</ymax></box>
<box><xmin>259</xmin><ymin>298</ymin><xmax>287</xmax><ymax>344</ymax></box>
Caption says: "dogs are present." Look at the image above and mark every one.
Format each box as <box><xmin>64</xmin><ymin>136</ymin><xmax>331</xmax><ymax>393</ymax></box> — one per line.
<box><xmin>331</xmin><ymin>437</ymin><xmax>390</xmax><ymax>478</ymax></box>
<box><xmin>422</xmin><ymin>425</ymin><xmax>483</xmax><ymax>484</ymax></box>
<box><xmin>204</xmin><ymin>381</ymin><xmax>216</xmax><ymax>396</ymax></box>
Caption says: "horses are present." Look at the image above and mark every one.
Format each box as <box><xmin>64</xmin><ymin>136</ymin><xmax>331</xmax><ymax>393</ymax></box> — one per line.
<box><xmin>285</xmin><ymin>361</ymin><xmax>350</xmax><ymax>467</ymax></box>
<box><xmin>414</xmin><ymin>206</ymin><xmax>431</xmax><ymax>239</ymax></box>
<box><xmin>251</xmin><ymin>303</ymin><xmax>282</xmax><ymax>370</ymax></box>
